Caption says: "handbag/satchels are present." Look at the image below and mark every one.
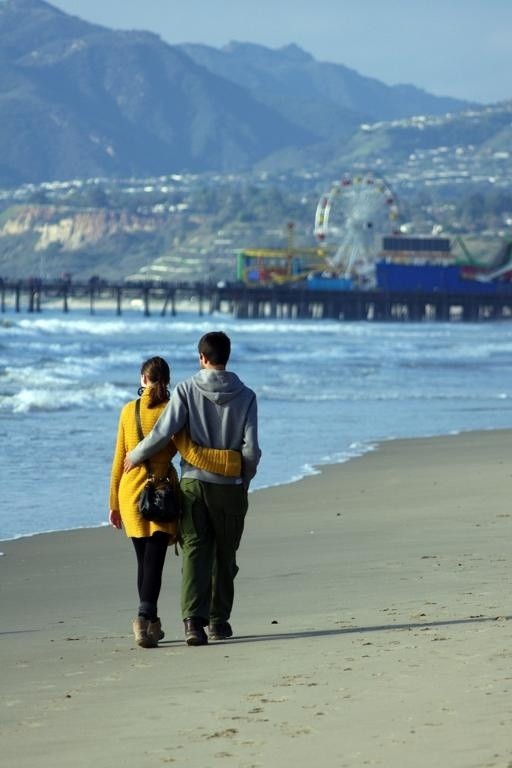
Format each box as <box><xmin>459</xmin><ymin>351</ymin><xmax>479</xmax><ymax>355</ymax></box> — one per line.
<box><xmin>139</xmin><ymin>477</ymin><xmax>182</xmax><ymax>523</ymax></box>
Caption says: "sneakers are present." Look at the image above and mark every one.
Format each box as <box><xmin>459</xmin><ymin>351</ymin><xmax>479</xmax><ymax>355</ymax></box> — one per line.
<box><xmin>131</xmin><ymin>617</ymin><xmax>159</xmax><ymax>649</ymax></box>
<box><xmin>183</xmin><ymin>617</ymin><xmax>208</xmax><ymax>646</ymax></box>
<box><xmin>208</xmin><ymin>619</ymin><xmax>233</xmax><ymax>640</ymax></box>
<box><xmin>150</xmin><ymin>617</ymin><xmax>164</xmax><ymax>641</ymax></box>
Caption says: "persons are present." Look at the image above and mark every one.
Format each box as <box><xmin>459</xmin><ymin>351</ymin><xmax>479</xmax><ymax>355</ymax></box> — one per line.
<box><xmin>123</xmin><ymin>329</ymin><xmax>262</xmax><ymax>646</ymax></box>
<box><xmin>108</xmin><ymin>355</ymin><xmax>242</xmax><ymax>647</ymax></box>
